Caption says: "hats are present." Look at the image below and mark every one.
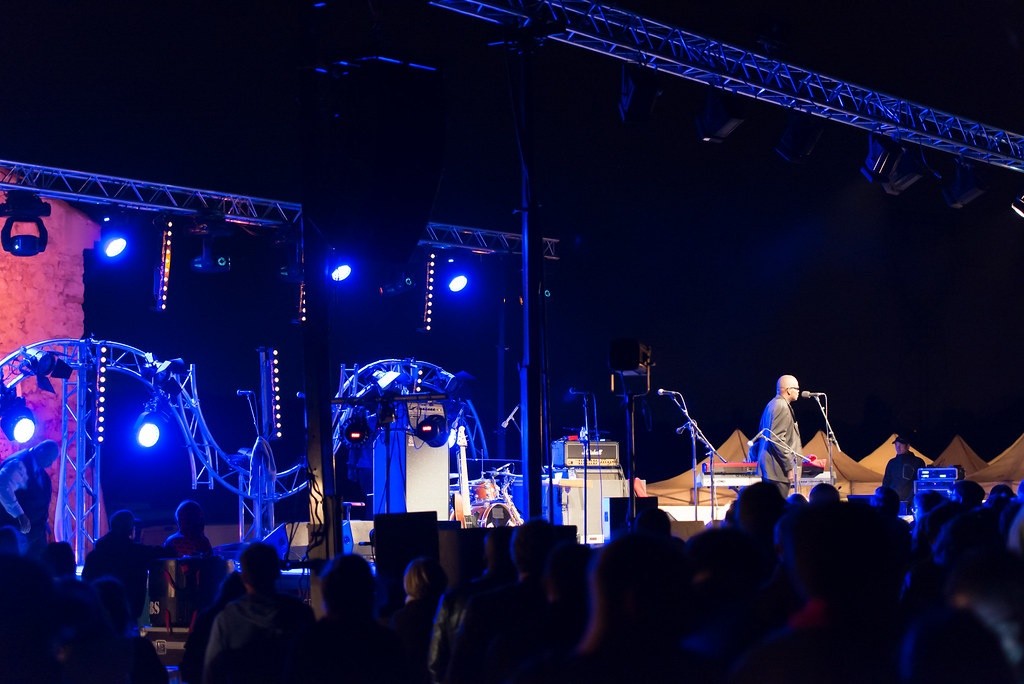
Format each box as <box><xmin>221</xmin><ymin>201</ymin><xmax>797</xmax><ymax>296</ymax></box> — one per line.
<box><xmin>892</xmin><ymin>437</ymin><xmax>910</xmax><ymax>444</ymax></box>
<box><xmin>110</xmin><ymin>509</ymin><xmax>135</xmax><ymax>532</ymax></box>
<box><xmin>635</xmin><ymin>509</ymin><xmax>668</xmax><ymax>532</ymax></box>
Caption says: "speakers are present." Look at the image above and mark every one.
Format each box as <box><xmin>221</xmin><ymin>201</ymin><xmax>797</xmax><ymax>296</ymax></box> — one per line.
<box><xmin>552</xmin><ymin>467</ymin><xmax>626</xmax><ymax>509</ymax></box>
<box><xmin>262</xmin><ymin>522</ymin><xmax>311</xmax><ymax>565</ymax></box>
<box><xmin>373</xmin><ymin>413</ymin><xmax>449</xmax><ymax>521</ymax></box>
<box><xmin>342</xmin><ymin>519</ymin><xmax>376</xmax><ymax>557</ymax></box>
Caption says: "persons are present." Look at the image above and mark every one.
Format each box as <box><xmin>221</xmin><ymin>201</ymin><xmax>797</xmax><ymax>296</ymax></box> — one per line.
<box><xmin>0</xmin><ymin>481</ymin><xmax>1024</xmax><ymax>684</ymax></box>
<box><xmin>952</xmin><ymin>465</ymin><xmax>966</xmax><ymax>482</ymax></box>
<box><xmin>0</xmin><ymin>439</ymin><xmax>59</xmax><ymax>545</ymax></box>
<box><xmin>881</xmin><ymin>435</ymin><xmax>925</xmax><ymax>502</ymax></box>
<box><xmin>754</xmin><ymin>375</ymin><xmax>802</xmax><ymax>498</ymax></box>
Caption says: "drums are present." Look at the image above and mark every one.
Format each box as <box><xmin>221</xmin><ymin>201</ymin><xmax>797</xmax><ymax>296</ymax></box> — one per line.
<box><xmin>450</xmin><ymin>502</ymin><xmax>515</xmax><ymax>528</ymax></box>
<box><xmin>472</xmin><ymin>479</ymin><xmax>498</xmax><ymax>503</ymax></box>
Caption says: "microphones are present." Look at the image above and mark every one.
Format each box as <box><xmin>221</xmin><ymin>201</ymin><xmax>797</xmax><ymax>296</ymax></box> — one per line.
<box><xmin>296</xmin><ymin>392</ymin><xmax>306</xmax><ymax>398</ymax></box>
<box><xmin>747</xmin><ymin>428</ymin><xmax>765</xmax><ymax>446</ymax></box>
<box><xmin>357</xmin><ymin>541</ymin><xmax>373</xmax><ymax>546</ymax></box>
<box><xmin>236</xmin><ymin>389</ymin><xmax>253</xmax><ymax>396</ymax></box>
<box><xmin>501</xmin><ymin>406</ymin><xmax>519</xmax><ymax>428</ymax></box>
<box><xmin>801</xmin><ymin>391</ymin><xmax>825</xmax><ymax>398</ymax></box>
<box><xmin>657</xmin><ymin>389</ymin><xmax>679</xmax><ymax>395</ymax></box>
<box><xmin>451</xmin><ymin>406</ymin><xmax>465</xmax><ymax>428</ymax></box>
<box><xmin>676</xmin><ymin>421</ymin><xmax>690</xmax><ymax>434</ymax></box>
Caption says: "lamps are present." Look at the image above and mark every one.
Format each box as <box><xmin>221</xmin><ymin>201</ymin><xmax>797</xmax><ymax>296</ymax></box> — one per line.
<box><xmin>338</xmin><ymin>368</ymin><xmax>478</xmax><ymax>447</ymax></box>
<box><xmin>443</xmin><ymin>259</ymin><xmax>467</xmax><ymax>292</ymax></box>
<box><xmin>101</xmin><ymin>218</ymin><xmax>126</xmax><ymax>259</ymax></box>
<box><xmin>144</xmin><ymin>352</ymin><xmax>184</xmax><ymax>401</ymax></box>
<box><xmin>1</xmin><ymin>396</ymin><xmax>36</xmax><ymax>446</ymax></box>
<box><xmin>326</xmin><ymin>246</ymin><xmax>352</xmax><ymax>282</ymax></box>
<box><xmin>0</xmin><ymin>188</ymin><xmax>51</xmax><ymax>257</ymax></box>
<box><xmin>12</xmin><ymin>341</ymin><xmax>73</xmax><ymax>392</ymax></box>
<box><xmin>136</xmin><ymin>405</ymin><xmax>160</xmax><ymax>449</ymax></box>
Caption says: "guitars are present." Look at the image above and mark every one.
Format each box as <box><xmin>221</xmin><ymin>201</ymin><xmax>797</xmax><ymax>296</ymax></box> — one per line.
<box><xmin>456</xmin><ymin>425</ymin><xmax>473</xmax><ymax>530</ymax></box>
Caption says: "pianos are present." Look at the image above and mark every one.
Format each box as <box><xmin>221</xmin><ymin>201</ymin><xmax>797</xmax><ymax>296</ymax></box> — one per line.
<box><xmin>700</xmin><ymin>458</ymin><xmax>837</xmax><ymax>518</ymax></box>
